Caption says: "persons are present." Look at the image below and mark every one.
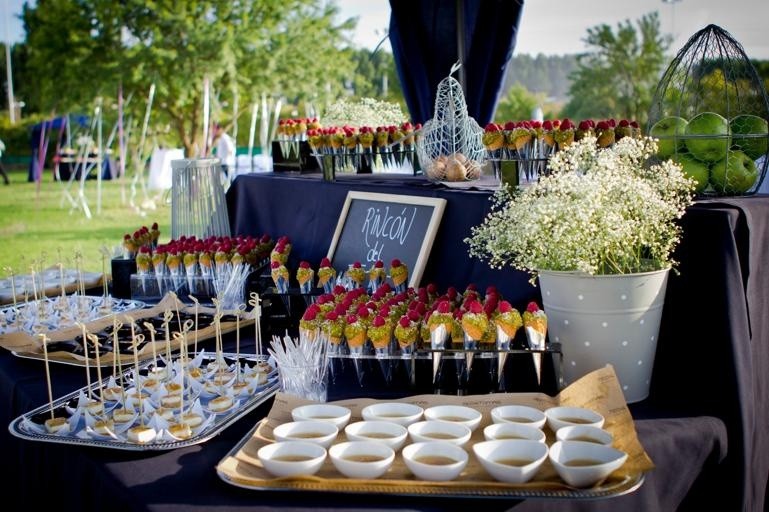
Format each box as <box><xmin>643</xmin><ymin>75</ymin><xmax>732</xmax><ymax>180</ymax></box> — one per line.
<box><xmin>0</xmin><ymin>139</ymin><xmax>11</xmax><ymax>184</ymax></box>
<box><xmin>214</xmin><ymin>122</ymin><xmax>239</xmax><ymax>185</ymax></box>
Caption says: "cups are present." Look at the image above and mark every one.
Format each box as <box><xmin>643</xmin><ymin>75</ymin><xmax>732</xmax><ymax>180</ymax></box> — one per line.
<box><xmin>277</xmin><ymin>356</ymin><xmax>330</xmax><ymax>404</ymax></box>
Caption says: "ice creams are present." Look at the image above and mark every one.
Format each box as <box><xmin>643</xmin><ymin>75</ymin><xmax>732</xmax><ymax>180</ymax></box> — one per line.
<box><xmin>120</xmin><ymin>218</ymin><xmax>271</xmax><ymax>299</ymax></box>
<box><xmin>290</xmin><ymin>256</ymin><xmax>413</xmax><ymax>302</ymax></box>
<box><xmin>275</xmin><ymin>114</ymin><xmax>319</xmax><ymax>161</ymax></box>
<box><xmin>483</xmin><ymin>117</ymin><xmax>642</xmax><ymax>190</ymax></box>
<box><xmin>267</xmin><ymin>235</ymin><xmax>290</xmax><ymax>303</ymax></box>
<box><xmin>302</xmin><ymin>121</ymin><xmax>423</xmax><ymax>185</ymax></box>
<box><xmin>296</xmin><ymin>284</ymin><xmax>551</xmax><ymax>391</ymax></box>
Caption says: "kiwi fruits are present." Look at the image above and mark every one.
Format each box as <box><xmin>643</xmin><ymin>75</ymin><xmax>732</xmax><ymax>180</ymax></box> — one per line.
<box><xmin>426</xmin><ymin>155</ymin><xmax>482</xmax><ymax>182</ymax></box>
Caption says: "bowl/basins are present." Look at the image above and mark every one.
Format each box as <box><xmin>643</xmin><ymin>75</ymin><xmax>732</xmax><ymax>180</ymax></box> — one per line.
<box><xmin>549</xmin><ymin>440</ymin><xmax>629</xmax><ymax>488</ymax></box>
<box><xmin>360</xmin><ymin>402</ymin><xmax>424</xmax><ymax>428</ymax></box>
<box><xmin>271</xmin><ymin>420</ymin><xmax>339</xmax><ymax>451</ymax></box>
<box><xmin>328</xmin><ymin>442</ymin><xmax>396</xmax><ymax>480</ymax></box>
<box><xmin>472</xmin><ymin>439</ymin><xmax>550</xmax><ymax>483</ymax></box>
<box><xmin>290</xmin><ymin>404</ymin><xmax>352</xmax><ymax>433</ymax></box>
<box><xmin>554</xmin><ymin>426</ymin><xmax>614</xmax><ymax>450</ymax></box>
<box><xmin>406</xmin><ymin>421</ymin><xmax>472</xmax><ymax>451</ymax></box>
<box><xmin>257</xmin><ymin>441</ymin><xmax>328</xmax><ymax>479</ymax></box>
<box><xmin>546</xmin><ymin>406</ymin><xmax>605</xmax><ymax>433</ymax></box>
<box><xmin>400</xmin><ymin>440</ymin><xmax>469</xmax><ymax>482</ymax></box>
<box><xmin>482</xmin><ymin>423</ymin><xmax>546</xmax><ymax>445</ymax></box>
<box><xmin>423</xmin><ymin>404</ymin><xmax>482</xmax><ymax>434</ymax></box>
<box><xmin>344</xmin><ymin>419</ymin><xmax>408</xmax><ymax>454</ymax></box>
<box><xmin>491</xmin><ymin>405</ymin><xmax>546</xmax><ymax>431</ymax></box>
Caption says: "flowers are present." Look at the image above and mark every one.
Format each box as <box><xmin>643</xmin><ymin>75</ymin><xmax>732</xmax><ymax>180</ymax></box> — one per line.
<box><xmin>463</xmin><ymin>132</ymin><xmax>699</xmax><ymax>288</ymax></box>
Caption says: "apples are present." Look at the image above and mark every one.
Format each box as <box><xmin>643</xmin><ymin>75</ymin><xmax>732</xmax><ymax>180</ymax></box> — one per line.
<box><xmin>664</xmin><ymin>152</ymin><xmax>711</xmax><ymax>196</ymax></box>
<box><xmin>708</xmin><ymin>147</ymin><xmax>759</xmax><ymax>197</ymax></box>
<box><xmin>646</xmin><ymin>115</ymin><xmax>690</xmax><ymax>159</ymax></box>
<box><xmin>729</xmin><ymin>114</ymin><xmax>769</xmax><ymax>161</ymax></box>
<box><xmin>685</xmin><ymin>112</ymin><xmax>733</xmax><ymax>165</ymax></box>
